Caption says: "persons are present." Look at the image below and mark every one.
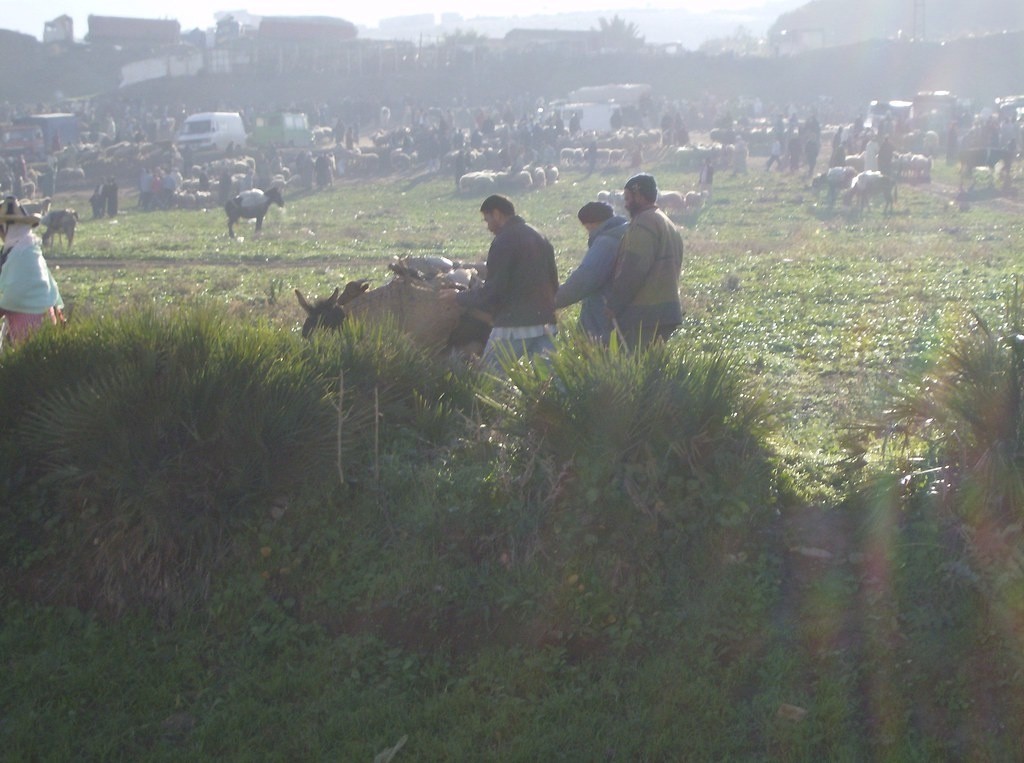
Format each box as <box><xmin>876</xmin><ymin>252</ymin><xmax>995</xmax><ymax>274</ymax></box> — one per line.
<box><xmin>602</xmin><ymin>174</ymin><xmax>684</xmax><ymax>353</ymax></box>
<box><xmin>90</xmin><ymin>175</ymin><xmax>119</xmax><ymax>219</ymax></box>
<box><xmin>1</xmin><ymin>90</ymin><xmax>1024</xmax><ymax>210</ymax></box>
<box><xmin>554</xmin><ymin>202</ymin><xmax>630</xmax><ymax>349</ymax></box>
<box><xmin>437</xmin><ymin>194</ymin><xmax>557</xmax><ymax>378</ymax></box>
<box><xmin>0</xmin><ymin>196</ymin><xmax>65</xmax><ymax>346</ymax></box>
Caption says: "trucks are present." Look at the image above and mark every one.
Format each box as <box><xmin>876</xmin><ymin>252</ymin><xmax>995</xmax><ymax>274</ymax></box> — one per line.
<box><xmin>0</xmin><ymin>112</ymin><xmax>76</xmax><ymax>162</ymax></box>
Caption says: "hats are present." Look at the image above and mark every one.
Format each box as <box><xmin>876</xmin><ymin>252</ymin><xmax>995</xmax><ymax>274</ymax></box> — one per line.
<box><xmin>578</xmin><ymin>201</ymin><xmax>614</xmax><ymax>223</ymax></box>
<box><xmin>624</xmin><ymin>174</ymin><xmax>657</xmax><ymax>209</ymax></box>
<box><xmin>0</xmin><ymin>195</ymin><xmax>41</xmax><ymax>227</ymax></box>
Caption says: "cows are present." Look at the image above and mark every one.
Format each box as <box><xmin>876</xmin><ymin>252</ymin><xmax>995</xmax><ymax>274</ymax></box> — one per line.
<box><xmin>295</xmin><ymin>277</ymin><xmax>468</xmax><ymax>363</ymax></box>
<box><xmin>0</xmin><ymin>125</ymin><xmax>1024</xmax><ymax>292</ymax></box>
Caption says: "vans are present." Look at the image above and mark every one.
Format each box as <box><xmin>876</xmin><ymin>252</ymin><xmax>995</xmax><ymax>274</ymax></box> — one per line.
<box><xmin>178</xmin><ymin>112</ymin><xmax>247</xmax><ymax>154</ymax></box>
<box><xmin>865</xmin><ymin>100</ymin><xmax>913</xmax><ymax>131</ymax></box>
<box><xmin>537</xmin><ymin>102</ymin><xmax>619</xmax><ymax>132</ymax></box>
